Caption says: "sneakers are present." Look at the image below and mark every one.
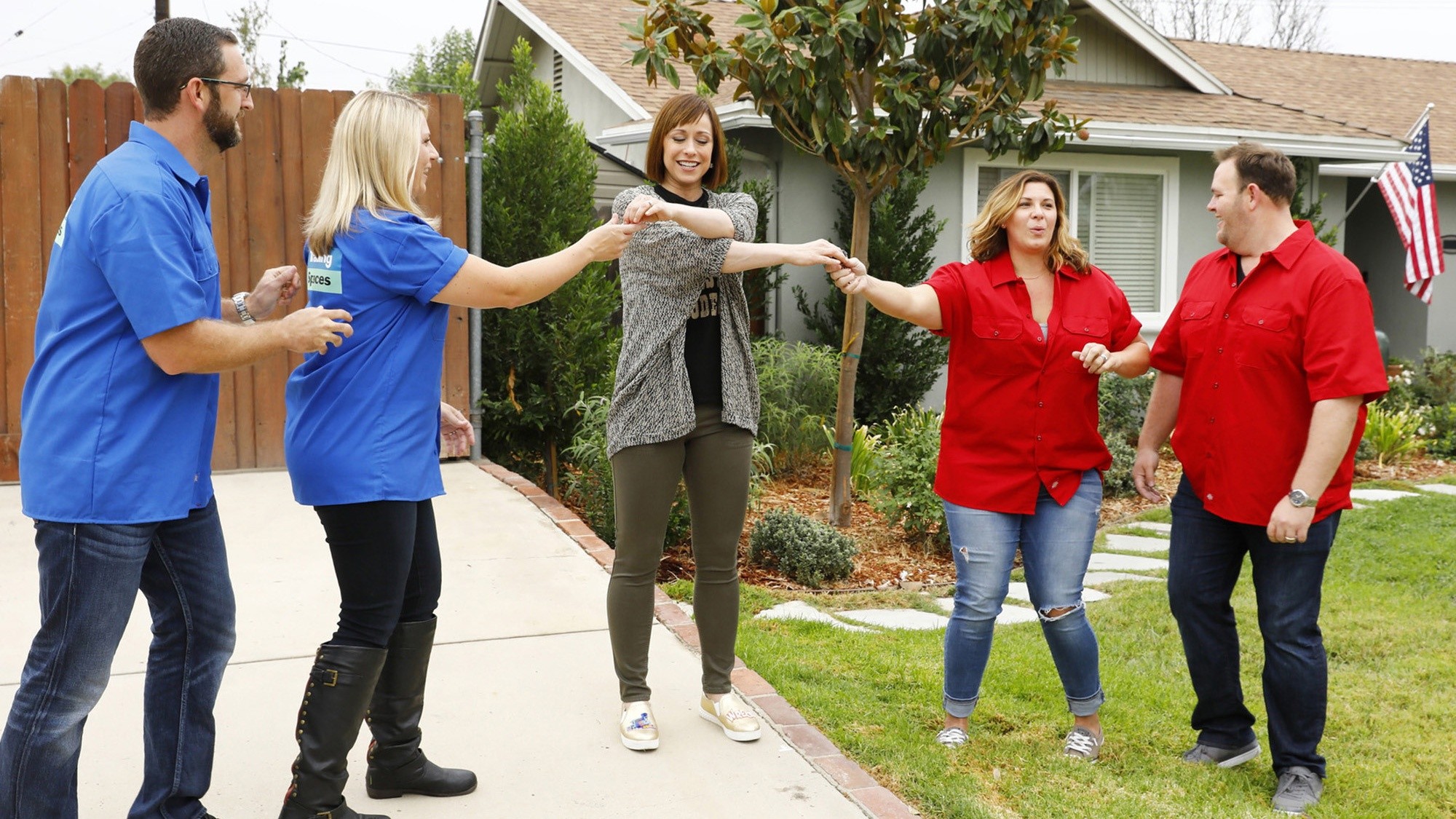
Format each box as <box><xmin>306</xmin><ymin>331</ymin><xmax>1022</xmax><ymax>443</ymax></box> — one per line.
<box><xmin>1063</xmin><ymin>726</ymin><xmax>1105</xmax><ymax>764</ymax></box>
<box><xmin>1182</xmin><ymin>738</ymin><xmax>1261</xmax><ymax>769</ymax></box>
<box><xmin>1271</xmin><ymin>766</ymin><xmax>1322</xmax><ymax>815</ymax></box>
<box><xmin>936</xmin><ymin>726</ymin><xmax>968</xmax><ymax>749</ymax></box>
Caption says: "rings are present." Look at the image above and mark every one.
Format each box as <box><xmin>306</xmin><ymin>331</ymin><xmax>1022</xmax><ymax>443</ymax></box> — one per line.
<box><xmin>1285</xmin><ymin>537</ymin><xmax>1296</xmax><ymax>544</ymax></box>
<box><xmin>1101</xmin><ymin>354</ymin><xmax>1106</xmax><ymax>360</ymax></box>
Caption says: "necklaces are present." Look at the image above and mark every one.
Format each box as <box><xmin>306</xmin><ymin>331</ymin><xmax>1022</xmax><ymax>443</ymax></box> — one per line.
<box><xmin>1017</xmin><ymin>269</ymin><xmax>1048</xmax><ymax>280</ymax></box>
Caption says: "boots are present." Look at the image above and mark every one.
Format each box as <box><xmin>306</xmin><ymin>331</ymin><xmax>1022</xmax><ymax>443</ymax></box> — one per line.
<box><xmin>278</xmin><ymin>641</ymin><xmax>390</xmax><ymax>819</ymax></box>
<box><xmin>365</xmin><ymin>613</ymin><xmax>477</xmax><ymax>799</ymax></box>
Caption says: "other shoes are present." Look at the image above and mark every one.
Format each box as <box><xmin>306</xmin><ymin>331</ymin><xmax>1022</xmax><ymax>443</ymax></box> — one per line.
<box><xmin>698</xmin><ymin>692</ymin><xmax>761</xmax><ymax>741</ymax></box>
<box><xmin>619</xmin><ymin>700</ymin><xmax>660</xmax><ymax>750</ymax></box>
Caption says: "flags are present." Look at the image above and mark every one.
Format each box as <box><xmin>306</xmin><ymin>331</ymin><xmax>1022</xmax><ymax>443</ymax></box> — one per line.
<box><xmin>1370</xmin><ymin>109</ymin><xmax>1445</xmax><ymax>305</ymax></box>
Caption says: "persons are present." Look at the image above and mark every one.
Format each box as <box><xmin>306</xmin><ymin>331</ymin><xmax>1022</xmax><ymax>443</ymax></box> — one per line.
<box><xmin>275</xmin><ymin>90</ymin><xmax>648</xmax><ymax>818</ymax></box>
<box><xmin>607</xmin><ymin>93</ymin><xmax>849</xmax><ymax>752</ymax></box>
<box><xmin>1</xmin><ymin>20</ymin><xmax>354</xmax><ymax>819</ymax></box>
<box><xmin>1131</xmin><ymin>142</ymin><xmax>1390</xmax><ymax>817</ymax></box>
<box><xmin>823</xmin><ymin>170</ymin><xmax>1150</xmax><ymax>763</ymax></box>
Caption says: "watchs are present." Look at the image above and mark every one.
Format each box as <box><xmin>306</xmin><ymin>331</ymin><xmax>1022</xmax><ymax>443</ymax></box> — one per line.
<box><xmin>1287</xmin><ymin>490</ymin><xmax>1318</xmax><ymax>509</ymax></box>
<box><xmin>232</xmin><ymin>291</ymin><xmax>256</xmax><ymax>324</ymax></box>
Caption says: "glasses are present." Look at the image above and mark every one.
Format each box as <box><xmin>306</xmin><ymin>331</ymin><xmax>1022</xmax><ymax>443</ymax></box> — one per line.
<box><xmin>178</xmin><ymin>77</ymin><xmax>252</xmax><ymax>98</ymax></box>
